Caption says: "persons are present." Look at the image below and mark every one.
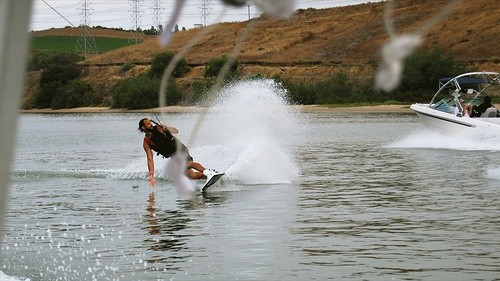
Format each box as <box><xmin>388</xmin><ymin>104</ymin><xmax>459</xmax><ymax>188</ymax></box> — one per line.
<box><xmin>138</xmin><ymin>117</ymin><xmax>219</xmax><ymax>188</ymax></box>
<box><xmin>455</xmin><ymin>96</ymin><xmax>500</xmax><ymax>118</ymax></box>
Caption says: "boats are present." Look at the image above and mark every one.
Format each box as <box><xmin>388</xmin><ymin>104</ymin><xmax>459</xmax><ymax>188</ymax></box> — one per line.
<box><xmin>411</xmin><ymin>70</ymin><xmax>500</xmax><ymax>150</ymax></box>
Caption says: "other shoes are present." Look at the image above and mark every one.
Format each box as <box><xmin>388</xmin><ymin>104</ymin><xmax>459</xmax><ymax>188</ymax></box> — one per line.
<box><xmin>202</xmin><ymin>168</ymin><xmax>219</xmax><ymax>183</ymax></box>
